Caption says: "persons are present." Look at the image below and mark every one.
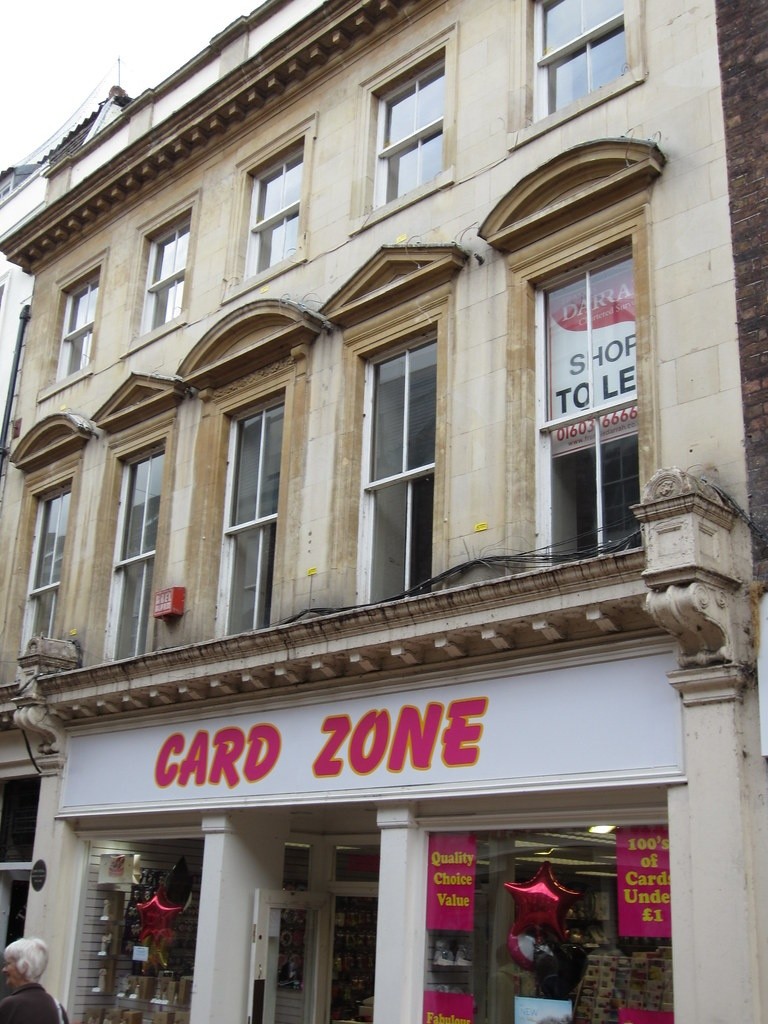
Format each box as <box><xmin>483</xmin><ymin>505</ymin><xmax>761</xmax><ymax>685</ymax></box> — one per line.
<box><xmin>0</xmin><ymin>937</ymin><xmax>71</xmax><ymax>1024</ymax></box>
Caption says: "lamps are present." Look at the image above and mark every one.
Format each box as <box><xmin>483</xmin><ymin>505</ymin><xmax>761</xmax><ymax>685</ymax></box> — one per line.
<box><xmin>96</xmin><ymin>897</ymin><xmax>114</xmax><ymax>993</ymax></box>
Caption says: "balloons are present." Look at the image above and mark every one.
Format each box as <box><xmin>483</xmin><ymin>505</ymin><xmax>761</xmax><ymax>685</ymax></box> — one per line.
<box><xmin>501</xmin><ymin>857</ymin><xmax>581</xmax><ymax>948</ymax></box>
<box><xmin>134</xmin><ymin>884</ymin><xmax>180</xmax><ymax>946</ymax></box>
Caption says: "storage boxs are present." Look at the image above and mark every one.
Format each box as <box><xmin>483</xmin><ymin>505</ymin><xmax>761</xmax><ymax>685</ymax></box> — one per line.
<box><xmin>83</xmin><ymin>974</ymin><xmax>193</xmax><ymax>1024</ymax></box>
